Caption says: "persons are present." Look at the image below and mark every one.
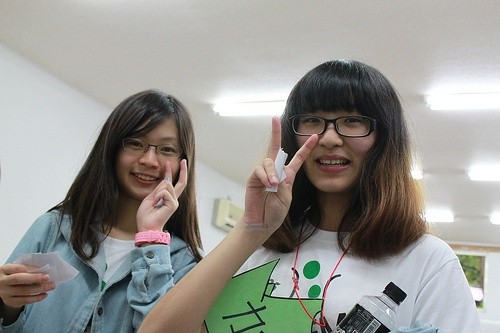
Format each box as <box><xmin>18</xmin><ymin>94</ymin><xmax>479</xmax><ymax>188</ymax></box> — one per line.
<box><xmin>136</xmin><ymin>59</ymin><xmax>486</xmax><ymax>333</ymax></box>
<box><xmin>0</xmin><ymin>89</ymin><xmax>206</xmax><ymax>333</ymax></box>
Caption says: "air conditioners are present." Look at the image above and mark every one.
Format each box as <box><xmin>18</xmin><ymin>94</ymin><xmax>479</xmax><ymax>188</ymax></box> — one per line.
<box><xmin>210</xmin><ymin>198</ymin><xmax>244</xmax><ymax>233</ymax></box>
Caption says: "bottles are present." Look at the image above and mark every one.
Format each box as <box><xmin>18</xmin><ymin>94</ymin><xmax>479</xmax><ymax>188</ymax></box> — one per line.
<box><xmin>330</xmin><ymin>282</ymin><xmax>407</xmax><ymax>333</ymax></box>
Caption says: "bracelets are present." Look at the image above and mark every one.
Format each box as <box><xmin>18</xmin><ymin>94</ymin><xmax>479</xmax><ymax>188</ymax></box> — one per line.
<box><xmin>134</xmin><ymin>230</ymin><xmax>172</xmax><ymax>247</ymax></box>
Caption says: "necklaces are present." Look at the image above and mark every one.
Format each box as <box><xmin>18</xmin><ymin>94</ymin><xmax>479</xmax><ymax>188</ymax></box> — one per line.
<box><xmin>285</xmin><ymin>199</ymin><xmax>364</xmax><ymax>333</ymax></box>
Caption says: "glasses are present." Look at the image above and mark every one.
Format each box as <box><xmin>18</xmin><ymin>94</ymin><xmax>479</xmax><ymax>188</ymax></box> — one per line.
<box><xmin>287</xmin><ymin>114</ymin><xmax>378</xmax><ymax>138</ymax></box>
<box><xmin>122</xmin><ymin>138</ymin><xmax>183</xmax><ymax>159</ymax></box>
